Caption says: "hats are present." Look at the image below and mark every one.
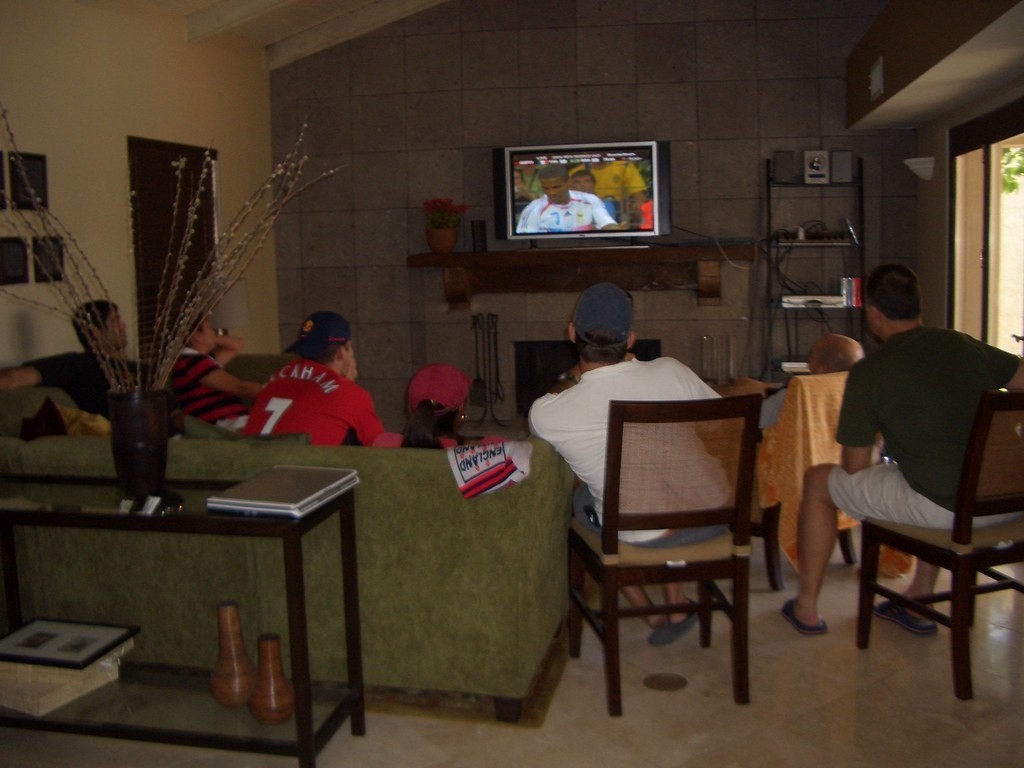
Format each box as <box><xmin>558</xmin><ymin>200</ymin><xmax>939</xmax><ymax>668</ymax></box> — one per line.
<box><xmin>407</xmin><ymin>362</ymin><xmax>474</xmax><ymax>417</ymax></box>
<box><xmin>284</xmin><ymin>310</ymin><xmax>351</xmax><ymax>361</ymax></box>
<box><xmin>571</xmin><ymin>282</ymin><xmax>634</xmax><ymax>348</ymax></box>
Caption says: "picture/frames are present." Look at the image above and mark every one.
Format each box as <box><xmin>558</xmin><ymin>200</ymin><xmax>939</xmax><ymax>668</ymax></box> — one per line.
<box><xmin>0</xmin><ymin>617</ymin><xmax>141</xmax><ymax>670</ymax></box>
<box><xmin>0</xmin><ymin>148</ymin><xmax>65</xmax><ymax>287</ymax></box>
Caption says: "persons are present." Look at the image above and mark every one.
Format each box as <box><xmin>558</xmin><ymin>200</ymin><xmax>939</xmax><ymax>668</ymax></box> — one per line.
<box><xmin>170</xmin><ymin>310</ymin><xmax>260</xmax><ymax>427</ymax></box>
<box><xmin>371</xmin><ymin>363</ymin><xmax>507</xmax><ymax>449</ymax></box>
<box><xmin>781</xmin><ymin>263</ymin><xmax>1024</xmax><ymax>635</ymax></box>
<box><xmin>241</xmin><ymin>310</ymin><xmax>385</xmax><ymax>446</ymax></box>
<box><xmin>742</xmin><ymin>334</ymin><xmax>866</xmax><ymax>428</ymax></box>
<box><xmin>514</xmin><ymin>152</ymin><xmax>647</xmax><ymax>232</ymax></box>
<box><xmin>528</xmin><ymin>283</ymin><xmax>724</xmax><ymax>643</ymax></box>
<box><xmin>0</xmin><ymin>301</ymin><xmax>147</xmax><ymax>421</ymax></box>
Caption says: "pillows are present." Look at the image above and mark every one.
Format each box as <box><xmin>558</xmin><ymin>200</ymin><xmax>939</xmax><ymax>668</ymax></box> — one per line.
<box><xmin>183</xmin><ymin>413</ymin><xmax>310</xmax><ymax>444</ymax></box>
<box><xmin>20</xmin><ymin>396</ymin><xmax>66</xmax><ymax>441</ymax></box>
<box><xmin>55</xmin><ymin>404</ymin><xmax>111</xmax><ymax>436</ymax></box>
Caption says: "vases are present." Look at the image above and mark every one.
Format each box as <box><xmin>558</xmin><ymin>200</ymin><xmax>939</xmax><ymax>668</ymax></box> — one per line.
<box><xmin>426</xmin><ymin>229</ymin><xmax>457</xmax><ymax>255</ymax></box>
<box><xmin>106</xmin><ymin>389</ymin><xmax>169</xmax><ymax>497</ymax></box>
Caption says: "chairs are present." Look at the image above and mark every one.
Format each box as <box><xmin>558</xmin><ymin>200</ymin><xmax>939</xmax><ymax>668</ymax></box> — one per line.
<box><xmin>569</xmin><ymin>392</ymin><xmax>763</xmax><ymax>716</ymax></box>
<box><xmin>749</xmin><ymin>371</ymin><xmax>856</xmax><ymax>591</ymax></box>
<box><xmin>857</xmin><ymin>388</ymin><xmax>1024</xmax><ymax>700</ymax></box>
<box><xmin>807</xmin><ymin>333</ymin><xmax>861</xmax><ymax>371</ymax></box>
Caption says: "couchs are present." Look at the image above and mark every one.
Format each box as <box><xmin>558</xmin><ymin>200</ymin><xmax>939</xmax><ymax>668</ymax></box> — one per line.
<box><xmin>0</xmin><ymin>353</ymin><xmax>575</xmax><ymax>722</ymax></box>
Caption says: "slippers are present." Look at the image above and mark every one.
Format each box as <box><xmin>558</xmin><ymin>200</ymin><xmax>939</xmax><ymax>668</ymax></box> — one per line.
<box><xmin>781</xmin><ymin>598</ymin><xmax>828</xmax><ymax>635</ymax></box>
<box><xmin>647</xmin><ymin>597</ymin><xmax>698</xmax><ymax>646</ymax></box>
<box><xmin>872</xmin><ymin>598</ymin><xmax>937</xmax><ymax>633</ymax></box>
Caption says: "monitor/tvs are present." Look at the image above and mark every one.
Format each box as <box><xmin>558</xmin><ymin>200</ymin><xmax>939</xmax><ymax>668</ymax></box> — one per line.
<box><xmin>493</xmin><ymin>140</ymin><xmax>672</xmax><ymax>240</ymax></box>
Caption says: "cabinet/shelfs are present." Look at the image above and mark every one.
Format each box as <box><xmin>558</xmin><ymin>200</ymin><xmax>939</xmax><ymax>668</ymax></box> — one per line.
<box><xmin>0</xmin><ymin>476</ymin><xmax>366</xmax><ymax>768</ymax></box>
<box><xmin>766</xmin><ymin>158</ymin><xmax>866</xmax><ymax>378</ymax></box>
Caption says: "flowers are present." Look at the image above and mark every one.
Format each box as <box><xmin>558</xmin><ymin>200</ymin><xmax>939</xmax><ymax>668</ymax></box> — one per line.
<box><xmin>423</xmin><ymin>199</ymin><xmax>466</xmax><ymax>229</ymax></box>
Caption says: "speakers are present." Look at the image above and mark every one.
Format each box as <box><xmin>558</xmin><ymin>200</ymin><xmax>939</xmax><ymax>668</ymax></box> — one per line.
<box><xmin>830</xmin><ymin>151</ymin><xmax>852</xmax><ymax>184</ymax></box>
<box><xmin>773</xmin><ymin>151</ymin><xmax>795</xmax><ymax>184</ymax></box>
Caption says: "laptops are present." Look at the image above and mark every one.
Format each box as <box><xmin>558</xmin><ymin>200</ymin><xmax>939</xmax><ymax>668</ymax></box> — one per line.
<box><xmin>207</xmin><ymin>466</ymin><xmax>359</xmax><ymax>518</ymax></box>
